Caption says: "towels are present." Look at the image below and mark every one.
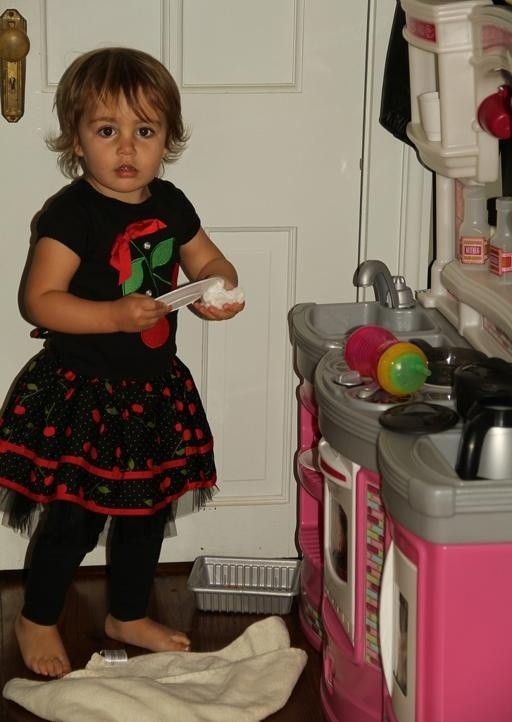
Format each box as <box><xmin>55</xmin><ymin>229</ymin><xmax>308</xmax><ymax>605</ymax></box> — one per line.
<box><xmin>4</xmin><ymin>615</ymin><xmax>310</xmax><ymax>722</ymax></box>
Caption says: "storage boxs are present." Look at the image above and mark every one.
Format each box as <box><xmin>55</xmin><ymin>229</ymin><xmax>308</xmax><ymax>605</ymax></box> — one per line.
<box><xmin>184</xmin><ymin>555</ymin><xmax>304</xmax><ymax>614</ymax></box>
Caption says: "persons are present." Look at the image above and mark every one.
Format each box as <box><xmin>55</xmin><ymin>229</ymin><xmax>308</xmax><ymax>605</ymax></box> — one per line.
<box><xmin>0</xmin><ymin>46</ymin><xmax>247</xmax><ymax>679</ymax></box>
